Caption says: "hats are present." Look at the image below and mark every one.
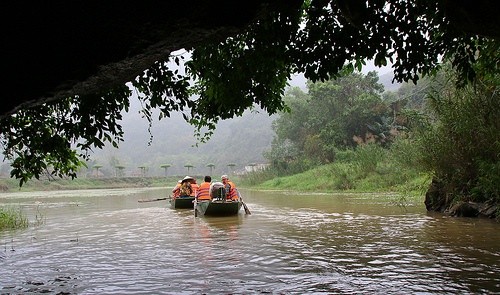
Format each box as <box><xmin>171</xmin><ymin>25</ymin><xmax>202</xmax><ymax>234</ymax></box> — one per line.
<box><xmin>182</xmin><ymin>176</ymin><xmax>193</xmax><ymax>182</ymax></box>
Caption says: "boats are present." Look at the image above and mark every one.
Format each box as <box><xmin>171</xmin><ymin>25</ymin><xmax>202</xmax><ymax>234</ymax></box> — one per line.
<box><xmin>169</xmin><ymin>195</ymin><xmax>196</xmax><ymax>210</ymax></box>
<box><xmin>193</xmin><ymin>198</ymin><xmax>244</xmax><ymax>217</ymax></box>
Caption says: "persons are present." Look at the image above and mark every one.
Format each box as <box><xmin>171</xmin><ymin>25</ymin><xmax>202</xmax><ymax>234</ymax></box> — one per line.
<box><xmin>197</xmin><ymin>176</ymin><xmax>212</xmax><ymax>201</ymax></box>
<box><xmin>222</xmin><ymin>175</ymin><xmax>238</xmax><ymax>201</ymax></box>
<box><xmin>171</xmin><ymin>176</ymin><xmax>199</xmax><ymax>198</ymax></box>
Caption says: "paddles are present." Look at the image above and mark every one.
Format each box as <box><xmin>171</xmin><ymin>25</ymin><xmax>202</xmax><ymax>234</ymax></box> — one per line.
<box><xmin>138</xmin><ymin>196</ymin><xmax>179</xmax><ymax>203</ymax></box>
<box><xmin>242</xmin><ymin>202</ymin><xmax>251</xmax><ymax>214</ymax></box>
<box><xmin>194</xmin><ymin>189</ymin><xmax>197</xmax><ymax>217</ymax></box>
